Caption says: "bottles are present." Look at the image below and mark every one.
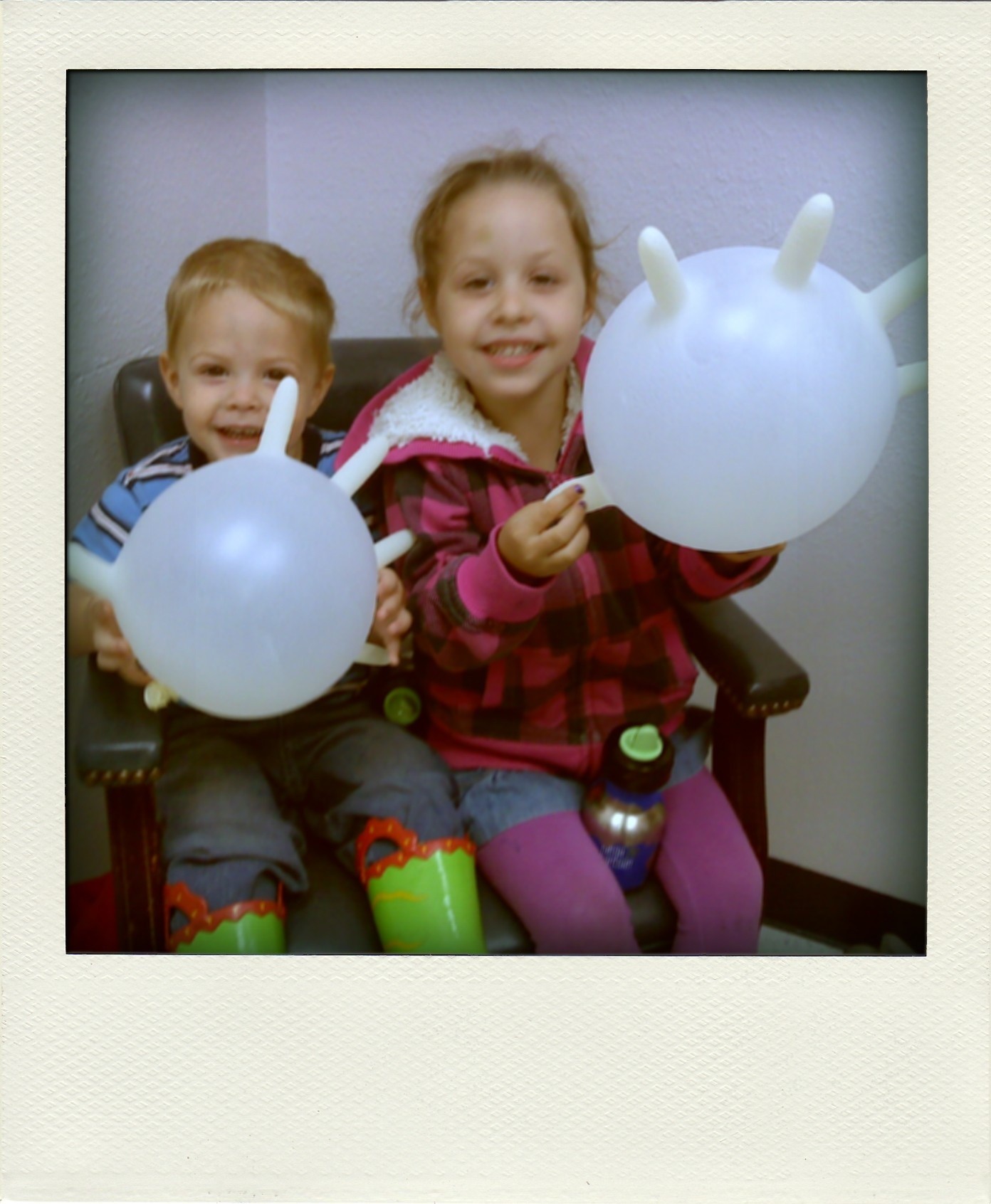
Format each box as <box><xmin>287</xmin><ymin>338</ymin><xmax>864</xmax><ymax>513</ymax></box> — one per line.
<box><xmin>581</xmin><ymin>723</ymin><xmax>676</xmax><ymax>894</ymax></box>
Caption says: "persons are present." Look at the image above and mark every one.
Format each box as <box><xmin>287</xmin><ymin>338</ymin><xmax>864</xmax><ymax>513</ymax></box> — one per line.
<box><xmin>331</xmin><ymin>149</ymin><xmax>786</xmax><ymax>957</ymax></box>
<box><xmin>64</xmin><ymin>241</ymin><xmax>485</xmax><ymax>961</ymax></box>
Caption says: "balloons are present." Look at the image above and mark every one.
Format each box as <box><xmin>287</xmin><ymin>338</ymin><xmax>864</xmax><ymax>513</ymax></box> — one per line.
<box><xmin>66</xmin><ymin>375</ymin><xmax>417</xmax><ymax>712</ymax></box>
<box><xmin>543</xmin><ymin>192</ymin><xmax>928</xmax><ymax>557</ymax></box>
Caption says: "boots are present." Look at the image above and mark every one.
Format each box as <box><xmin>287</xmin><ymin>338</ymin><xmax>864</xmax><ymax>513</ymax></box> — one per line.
<box><xmin>163</xmin><ymin>881</ymin><xmax>288</xmax><ymax>954</ymax></box>
<box><xmin>354</xmin><ymin>816</ymin><xmax>487</xmax><ymax>954</ymax></box>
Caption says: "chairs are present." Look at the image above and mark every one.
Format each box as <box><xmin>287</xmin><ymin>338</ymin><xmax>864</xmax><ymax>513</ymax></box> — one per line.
<box><xmin>74</xmin><ymin>332</ymin><xmax>812</xmax><ymax>953</ymax></box>
